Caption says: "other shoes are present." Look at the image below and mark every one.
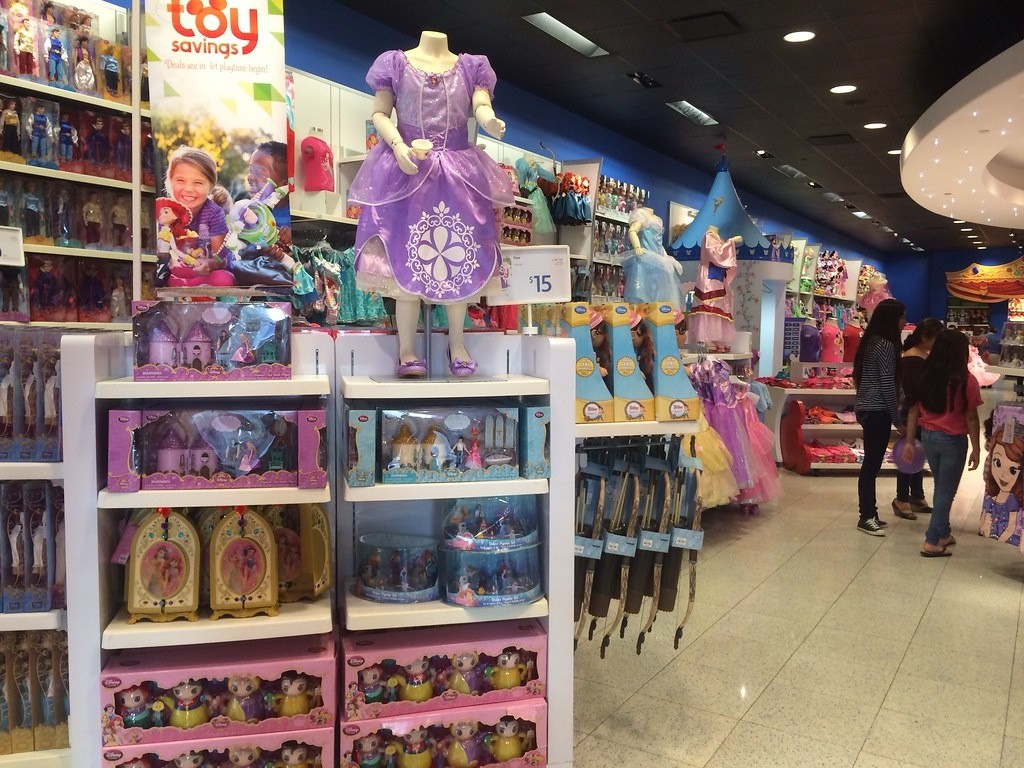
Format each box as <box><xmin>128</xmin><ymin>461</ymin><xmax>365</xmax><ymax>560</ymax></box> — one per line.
<box><xmin>447</xmin><ymin>341</ymin><xmax>477</xmax><ymax>377</ymax></box>
<box><xmin>892</xmin><ymin>498</ymin><xmax>917</xmax><ymax>520</ymax></box>
<box><xmin>910</xmin><ymin>499</ymin><xmax>934</xmax><ymax>513</ymax></box>
<box><xmin>396</xmin><ymin>355</ymin><xmax>428</xmax><ymax>376</ymax></box>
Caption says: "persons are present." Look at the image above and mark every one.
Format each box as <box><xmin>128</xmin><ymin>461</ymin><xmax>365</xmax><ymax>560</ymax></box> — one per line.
<box><xmin>466</xmin><ymin>439</ymin><xmax>481</xmax><ymax>469</ymax></box>
<box><xmin>356</xmin><ymin>650</ymin><xmax>528</xmax><ymax>768</ymax></box>
<box><xmin>217</xmin><ymin>331</ymin><xmax>254</xmax><ymax>362</ymax></box>
<box><xmin>820</xmin><ymin>317</ymin><xmax>843</xmax><ymax>362</ymax></box>
<box><xmin>689</xmin><ymin>225</ymin><xmax>743</xmax><ymax>351</ymax></box>
<box><xmin>122</xmin><ymin>670</ymin><xmax>321</xmax><ymax>768</ymax></box>
<box><xmin>516</xmin><ymin>154</ymin><xmax>561</xmax><ymax>233</ymax></box>
<box><xmin>238</xmin><ymin>141</ymin><xmax>291</xmax><ymax>260</ymax></box>
<box><xmin>585</xmin><ymin>306</ymin><xmax>691</xmax><ymax>376</ymax></box>
<box><xmin>541</xmin><ymin>172</ymin><xmax>590</xmax><ymax>226</ymax></box>
<box><xmin>478</xmin><ymin>519</ymin><xmax>488</xmax><ymax>536</ymax></box>
<box><xmin>624</xmin><ymin>206</ymin><xmax>684</xmax><ymax>307</ymax></box>
<box><xmin>400</xmin><ymin>566</ymin><xmax>407</xmax><ymax>581</ymax></box>
<box><xmin>226</xmin><ymin>438</ymin><xmax>261</xmax><ymax>471</ymax></box>
<box><xmin>802</xmin><ymin>250</ymin><xmax>848</xmax><ymax>293</ymax></box>
<box><xmin>451</xmin><ymin>437</ymin><xmax>471</xmax><ymax>467</ymax></box>
<box><xmin>800</xmin><ymin>319</ymin><xmax>820</xmax><ymax>362</ymax></box>
<box><xmin>591</xmin><ymin>174</ymin><xmax>645</xmax><ymax>295</ymax></box>
<box><xmin>349</xmin><ymin>31</ymin><xmax>507</xmax><ymax>377</ymax></box>
<box><xmin>854</xmin><ymin>299</ymin><xmax>906</xmax><ymax>535</ymax></box>
<box><xmin>0</xmin><ymin>0</ymin><xmax>154</xmax><ymax>319</ymax></box>
<box><xmin>892</xmin><ymin>317</ymin><xmax>943</xmax><ymax>520</ymax></box>
<box><xmin>861</xmin><ymin>275</ymin><xmax>888</xmax><ymax>321</ymax></box>
<box><xmin>167</xmin><ymin>149</ymin><xmax>235</xmax><ymax>269</ymax></box>
<box><xmin>902</xmin><ymin>332</ymin><xmax>982</xmax><ymax>556</ymax></box>
<box><xmin>842</xmin><ymin>319</ymin><xmax>863</xmax><ymax>362</ymax></box>
<box><xmin>502</xmin><ymin>569</ymin><xmax>507</xmax><ymax>588</ymax></box>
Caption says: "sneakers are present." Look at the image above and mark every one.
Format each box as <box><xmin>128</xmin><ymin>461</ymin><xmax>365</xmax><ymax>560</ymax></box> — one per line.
<box><xmin>878</xmin><ymin>520</ymin><xmax>888</xmax><ymax>528</ymax></box>
<box><xmin>857</xmin><ymin>515</ymin><xmax>882</xmax><ymax>535</ymax></box>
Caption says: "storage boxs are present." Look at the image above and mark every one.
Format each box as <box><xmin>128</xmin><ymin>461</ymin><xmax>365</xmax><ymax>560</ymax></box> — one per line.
<box><xmin>101</xmin><ymin>618</ymin><xmax>547</xmax><ymax>768</ymax></box>
<box><xmin>495</xmin><ymin>222</ymin><xmax>532</xmax><ymax>246</ymax></box>
<box><xmin>517</xmin><ymin>302</ymin><xmax>702</xmax><ymax>424</ymax></box>
<box><xmin>493</xmin><ymin>203</ymin><xmax>533</xmax><ymax>228</ymax></box>
<box><xmin>105</xmin><ymin>299</ymin><xmax>551</xmax><ymax>488</ymax></box>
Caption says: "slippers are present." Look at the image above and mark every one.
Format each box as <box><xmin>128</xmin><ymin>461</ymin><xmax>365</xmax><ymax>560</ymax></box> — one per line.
<box><xmin>920</xmin><ymin>547</ymin><xmax>952</xmax><ymax>557</ymax></box>
<box><xmin>943</xmin><ymin>536</ymin><xmax>955</xmax><ymax>546</ymax></box>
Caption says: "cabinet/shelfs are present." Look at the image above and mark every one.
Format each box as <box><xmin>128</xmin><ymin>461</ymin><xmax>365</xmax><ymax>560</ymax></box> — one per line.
<box><xmin>777</xmin><ymin>233</ymin><xmax>863</xmax><ymax>334</ymax></box>
<box><xmin>947</xmin><ymin>306</ymin><xmax>991</xmax><ymax>331</ymax></box>
<box><xmin>987</xmin><ymin>322</ymin><xmax>1024</xmax><ymax>396</ymax></box>
<box><xmin>285</xmin><ymin>63</ymin><xmax>561</xmax><ymax>250</ymax></box>
<box><xmin>763</xmin><ymin>362</ymin><xmax>930</xmax><ymax>475</ymax></box>
<box><xmin>0</xmin><ymin>332</ymin><xmax>576</xmax><ymax>768</ymax></box>
<box><xmin>1</xmin><ymin>1</ymin><xmax>158</xmax><ymax>333</ymax></box>
<box><xmin>559</xmin><ymin>158</ymin><xmax>630</xmax><ymax>306</ymax></box>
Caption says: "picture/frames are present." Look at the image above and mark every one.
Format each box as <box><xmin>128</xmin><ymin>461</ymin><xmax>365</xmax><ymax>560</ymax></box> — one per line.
<box><xmin>669</xmin><ymin>201</ymin><xmax>700</xmax><ymax>245</ymax></box>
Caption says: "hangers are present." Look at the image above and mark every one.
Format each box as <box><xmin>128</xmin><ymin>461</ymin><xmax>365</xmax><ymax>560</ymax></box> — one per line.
<box><xmin>290</xmin><ymin>230</ymin><xmax>357</xmax><ymax>254</ymax></box>
<box><xmin>697</xmin><ymin>341</ymin><xmax>708</xmax><ymax>365</ymax></box>
